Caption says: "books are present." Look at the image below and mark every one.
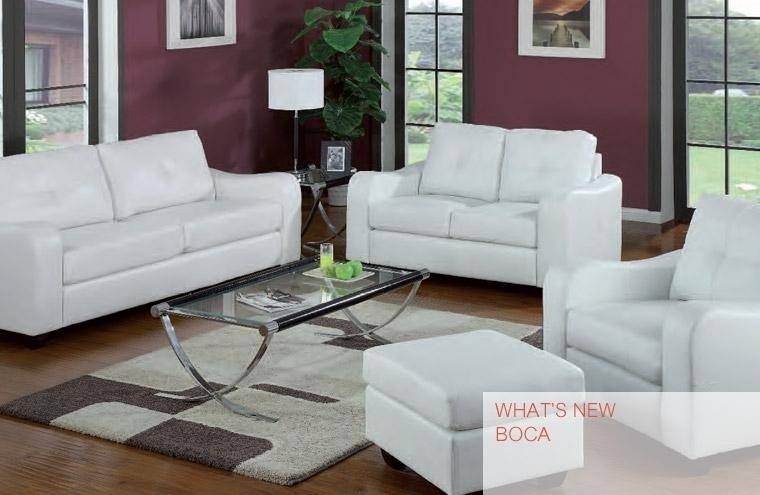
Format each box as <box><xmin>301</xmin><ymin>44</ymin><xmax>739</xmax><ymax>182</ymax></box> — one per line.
<box><xmin>235</xmin><ymin>287</ymin><xmax>307</xmax><ymax>314</ymax></box>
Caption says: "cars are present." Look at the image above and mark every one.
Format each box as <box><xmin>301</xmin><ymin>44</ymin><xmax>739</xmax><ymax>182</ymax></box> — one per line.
<box><xmin>712</xmin><ymin>86</ymin><xmax>759</xmax><ymax>98</ymax></box>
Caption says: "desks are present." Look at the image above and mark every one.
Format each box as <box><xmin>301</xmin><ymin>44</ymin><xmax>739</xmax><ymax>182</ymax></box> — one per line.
<box><xmin>292</xmin><ymin>167</ymin><xmax>352</xmax><ymax>254</ymax></box>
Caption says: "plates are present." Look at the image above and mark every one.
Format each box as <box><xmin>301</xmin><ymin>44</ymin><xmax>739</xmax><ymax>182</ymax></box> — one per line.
<box><xmin>301</xmin><ymin>266</ymin><xmax>375</xmax><ymax>283</ymax></box>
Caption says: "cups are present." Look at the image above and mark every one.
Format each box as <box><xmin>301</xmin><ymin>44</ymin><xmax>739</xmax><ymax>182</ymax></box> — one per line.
<box><xmin>319</xmin><ymin>242</ymin><xmax>334</xmax><ymax>277</ymax></box>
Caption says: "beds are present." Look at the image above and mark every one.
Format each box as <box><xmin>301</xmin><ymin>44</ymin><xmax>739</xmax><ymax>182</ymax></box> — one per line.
<box><xmin>145</xmin><ymin>259</ymin><xmax>430</xmax><ymax>418</ymax></box>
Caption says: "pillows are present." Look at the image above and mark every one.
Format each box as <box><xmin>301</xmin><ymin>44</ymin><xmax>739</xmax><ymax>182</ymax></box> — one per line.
<box><xmin>417</xmin><ymin>122</ymin><xmax>502</xmax><ymax>202</ymax></box>
<box><xmin>0</xmin><ymin>143</ymin><xmax>112</xmax><ymax>230</ymax></box>
<box><xmin>502</xmin><ymin>127</ymin><xmax>596</xmax><ymax>204</ymax></box>
<box><xmin>102</xmin><ymin>127</ymin><xmax>214</xmax><ymax>216</ymax></box>
<box><xmin>668</xmin><ymin>193</ymin><xmax>759</xmax><ymax>306</ymax></box>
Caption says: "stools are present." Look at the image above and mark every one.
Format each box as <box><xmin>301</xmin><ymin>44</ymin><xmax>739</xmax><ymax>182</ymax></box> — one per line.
<box><xmin>357</xmin><ymin>330</ymin><xmax>581</xmax><ymax>495</ymax></box>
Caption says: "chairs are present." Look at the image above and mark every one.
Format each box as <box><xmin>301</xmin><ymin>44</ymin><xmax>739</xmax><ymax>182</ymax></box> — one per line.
<box><xmin>537</xmin><ymin>204</ymin><xmax>759</xmax><ymax>462</ymax></box>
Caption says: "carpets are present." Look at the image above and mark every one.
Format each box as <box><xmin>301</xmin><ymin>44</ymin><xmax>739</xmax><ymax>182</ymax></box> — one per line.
<box><xmin>0</xmin><ymin>292</ymin><xmax>542</xmax><ymax>486</ymax></box>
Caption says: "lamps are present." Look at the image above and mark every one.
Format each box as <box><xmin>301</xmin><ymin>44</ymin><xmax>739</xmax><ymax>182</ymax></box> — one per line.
<box><xmin>267</xmin><ymin>68</ymin><xmax>324</xmax><ymax>179</ymax></box>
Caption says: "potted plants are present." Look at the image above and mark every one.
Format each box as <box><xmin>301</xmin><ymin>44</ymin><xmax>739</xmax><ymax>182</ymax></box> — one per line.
<box><xmin>290</xmin><ymin>0</ymin><xmax>389</xmax><ymax>208</ymax></box>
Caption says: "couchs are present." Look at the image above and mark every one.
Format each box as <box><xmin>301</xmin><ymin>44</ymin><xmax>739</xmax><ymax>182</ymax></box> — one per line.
<box><xmin>344</xmin><ymin>144</ymin><xmax>623</xmax><ymax>291</ymax></box>
<box><xmin>0</xmin><ymin>135</ymin><xmax>302</xmax><ymax>351</ymax></box>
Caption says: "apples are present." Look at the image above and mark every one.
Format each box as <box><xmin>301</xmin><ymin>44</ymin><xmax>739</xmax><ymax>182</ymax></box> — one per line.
<box><xmin>336</xmin><ymin>264</ymin><xmax>353</xmax><ymax>280</ymax></box>
<box><xmin>347</xmin><ymin>260</ymin><xmax>362</xmax><ymax>277</ymax></box>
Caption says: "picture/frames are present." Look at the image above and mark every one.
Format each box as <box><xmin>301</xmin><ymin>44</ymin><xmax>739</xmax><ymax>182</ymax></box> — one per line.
<box><xmin>515</xmin><ymin>1</ymin><xmax>608</xmax><ymax>62</ymax></box>
<box><xmin>165</xmin><ymin>0</ymin><xmax>237</xmax><ymax>52</ymax></box>
<box><xmin>318</xmin><ymin>138</ymin><xmax>352</xmax><ymax>175</ymax></box>
